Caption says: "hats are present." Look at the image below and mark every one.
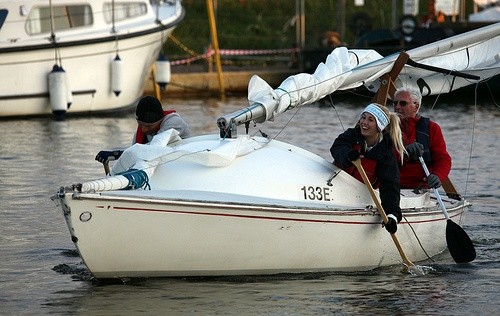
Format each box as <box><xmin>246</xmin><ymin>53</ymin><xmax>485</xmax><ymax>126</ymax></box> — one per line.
<box><xmin>136</xmin><ymin>96</ymin><xmax>164</xmax><ymax>125</ymax></box>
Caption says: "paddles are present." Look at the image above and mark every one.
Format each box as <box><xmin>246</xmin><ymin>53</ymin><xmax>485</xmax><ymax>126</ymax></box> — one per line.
<box><xmin>417</xmin><ymin>153</ymin><xmax>476</xmax><ymax>263</ymax></box>
<box><xmin>352</xmin><ymin>154</ymin><xmax>415</xmax><ymax>269</ymax></box>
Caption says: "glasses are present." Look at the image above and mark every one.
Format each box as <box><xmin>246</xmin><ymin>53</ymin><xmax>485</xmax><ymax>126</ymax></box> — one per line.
<box><xmin>390</xmin><ymin>101</ymin><xmax>416</xmax><ymax>107</ymax></box>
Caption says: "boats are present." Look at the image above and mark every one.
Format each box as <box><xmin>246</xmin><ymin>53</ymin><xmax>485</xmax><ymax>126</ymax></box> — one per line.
<box><xmin>0</xmin><ymin>0</ymin><xmax>185</xmax><ymax>118</ymax></box>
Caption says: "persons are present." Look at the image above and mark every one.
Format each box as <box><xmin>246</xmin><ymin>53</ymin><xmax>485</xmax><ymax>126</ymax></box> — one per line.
<box><xmin>351</xmin><ymin>11</ymin><xmax>387</xmax><ymax>48</ymax></box>
<box><xmin>131</xmin><ymin>96</ymin><xmax>191</xmax><ymax>147</ymax></box>
<box><xmin>393</xmin><ymin>85</ymin><xmax>452</xmax><ymax>189</ymax></box>
<box><xmin>330</xmin><ymin>103</ymin><xmax>403</xmax><ymax>234</ymax></box>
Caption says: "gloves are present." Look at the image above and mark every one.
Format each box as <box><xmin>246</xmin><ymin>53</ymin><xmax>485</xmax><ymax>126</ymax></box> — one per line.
<box><xmin>382</xmin><ymin>217</ymin><xmax>397</xmax><ymax>234</ymax></box>
<box><xmin>95</xmin><ymin>150</ymin><xmax>122</xmax><ymax>163</ymax></box>
<box><xmin>405</xmin><ymin>142</ymin><xmax>424</xmax><ymax>158</ymax></box>
<box><xmin>346</xmin><ymin>150</ymin><xmax>359</xmax><ymax>161</ymax></box>
<box><xmin>427</xmin><ymin>174</ymin><xmax>442</xmax><ymax>189</ymax></box>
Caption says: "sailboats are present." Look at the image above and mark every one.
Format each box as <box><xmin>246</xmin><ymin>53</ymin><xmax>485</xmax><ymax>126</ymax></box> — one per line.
<box><xmin>49</xmin><ymin>22</ymin><xmax>500</xmax><ymax>281</ymax></box>
